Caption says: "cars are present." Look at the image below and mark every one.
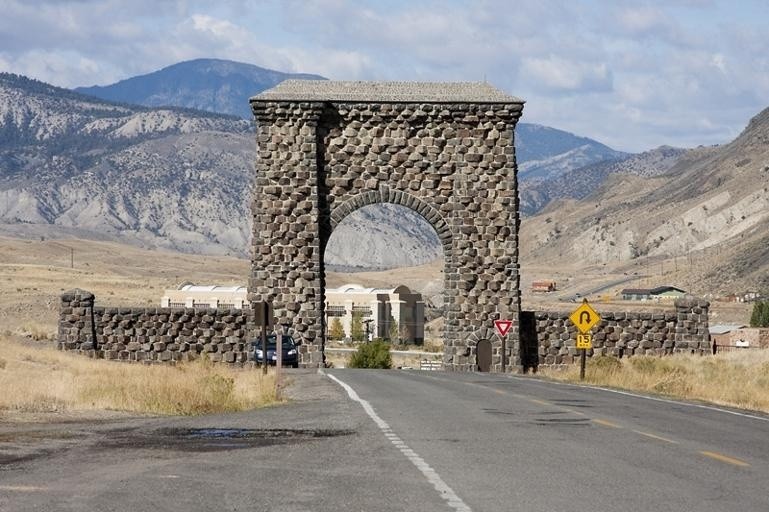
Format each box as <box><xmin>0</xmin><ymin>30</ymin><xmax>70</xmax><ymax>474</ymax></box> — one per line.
<box><xmin>250</xmin><ymin>334</ymin><xmax>302</xmax><ymax>369</ymax></box>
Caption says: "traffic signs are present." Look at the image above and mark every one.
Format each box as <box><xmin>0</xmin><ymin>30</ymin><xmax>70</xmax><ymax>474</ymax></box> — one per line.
<box><xmin>578</xmin><ymin>334</ymin><xmax>590</xmax><ymax>348</ymax></box>
